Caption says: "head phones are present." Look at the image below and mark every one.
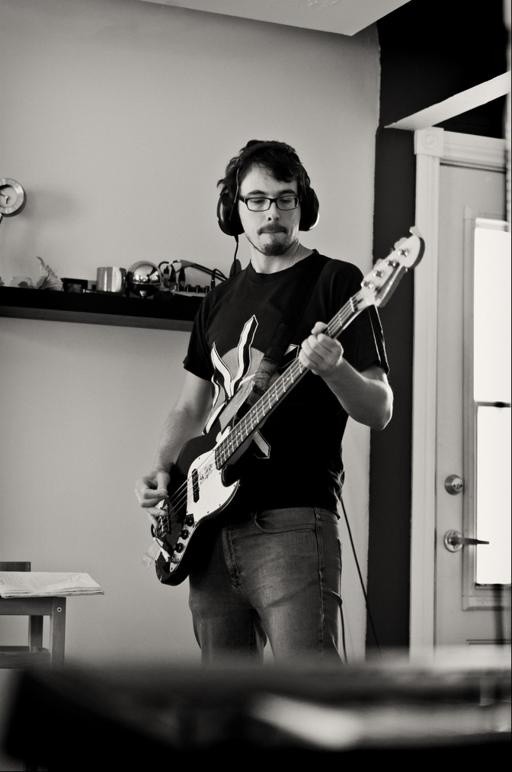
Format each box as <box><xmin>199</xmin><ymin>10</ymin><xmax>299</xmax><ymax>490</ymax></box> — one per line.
<box><xmin>217</xmin><ymin>154</ymin><xmax>320</xmax><ymax>237</ymax></box>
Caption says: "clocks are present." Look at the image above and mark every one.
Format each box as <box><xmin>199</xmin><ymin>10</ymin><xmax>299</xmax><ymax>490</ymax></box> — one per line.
<box><xmin>0</xmin><ymin>177</ymin><xmax>26</xmax><ymax>218</ymax></box>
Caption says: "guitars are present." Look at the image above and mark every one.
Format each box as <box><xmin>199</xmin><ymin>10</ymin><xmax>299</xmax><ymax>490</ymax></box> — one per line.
<box><xmin>151</xmin><ymin>232</ymin><xmax>424</xmax><ymax>586</ymax></box>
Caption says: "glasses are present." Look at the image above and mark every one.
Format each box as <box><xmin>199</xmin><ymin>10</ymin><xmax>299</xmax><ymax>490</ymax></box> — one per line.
<box><xmin>235</xmin><ymin>191</ymin><xmax>301</xmax><ymax>214</ymax></box>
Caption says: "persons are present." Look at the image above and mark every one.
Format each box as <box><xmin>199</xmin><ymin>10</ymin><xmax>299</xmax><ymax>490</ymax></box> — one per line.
<box><xmin>135</xmin><ymin>135</ymin><xmax>396</xmax><ymax>668</ymax></box>
